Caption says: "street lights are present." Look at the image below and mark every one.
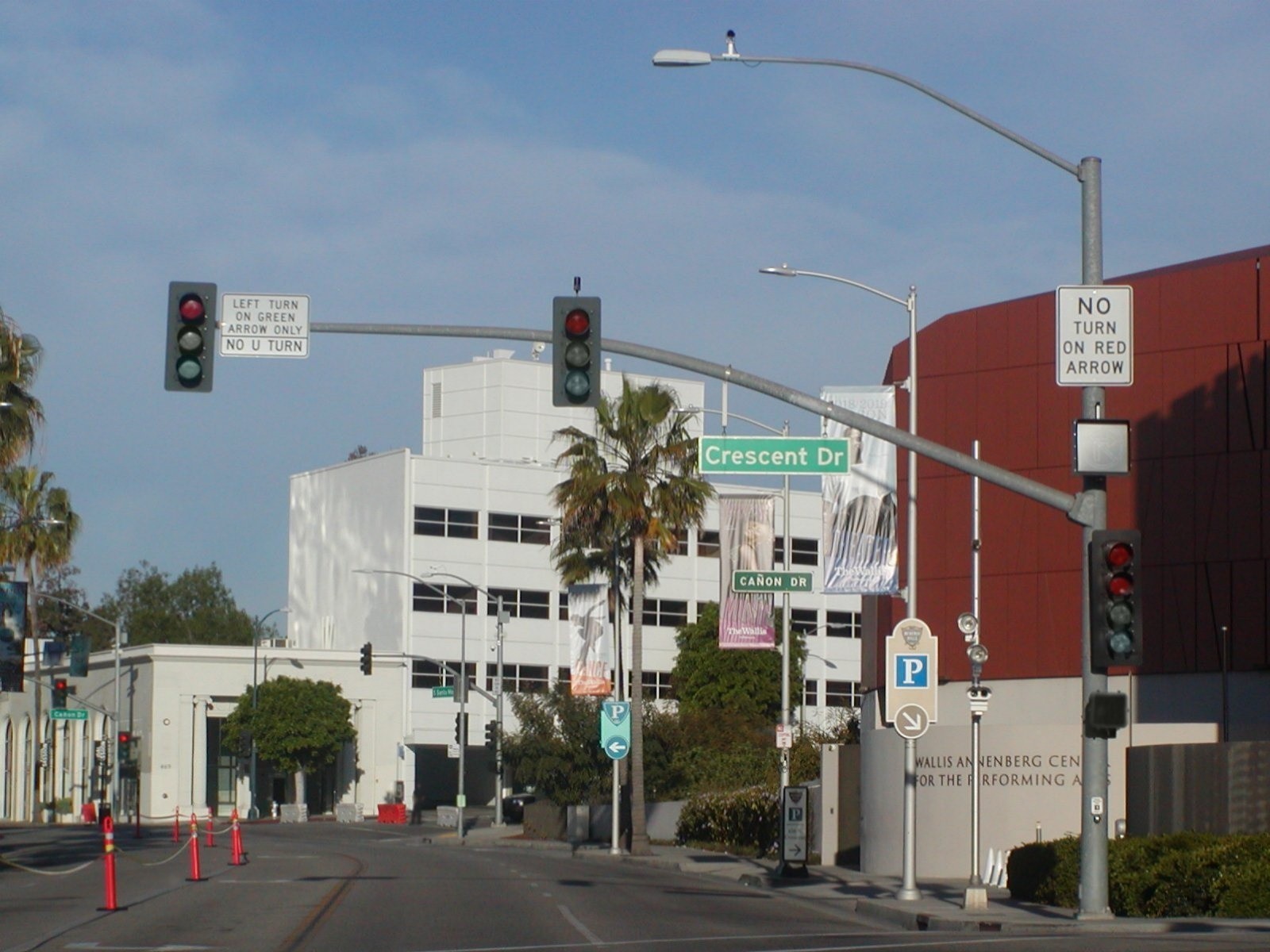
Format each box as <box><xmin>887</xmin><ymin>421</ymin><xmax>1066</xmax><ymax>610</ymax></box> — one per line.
<box><xmin>247</xmin><ymin>607</ymin><xmax>295</xmax><ymax>819</ymax></box>
<box><xmin>798</xmin><ymin>622</ymin><xmax>846</xmax><ymax>785</ymax></box>
<box><xmin>351</xmin><ymin>566</ymin><xmax>466</xmax><ymax>838</ymax></box>
<box><xmin>755</xmin><ymin>264</ymin><xmax>924</xmax><ymax>902</ymax></box>
<box><xmin>648</xmin><ymin>49</ymin><xmax>1115</xmax><ymax>923</ymax></box>
<box><xmin>419</xmin><ymin>571</ymin><xmax>506</xmax><ymax>830</ymax></box>
<box><xmin>670</xmin><ymin>405</ymin><xmax>793</xmax><ymax>877</ymax></box>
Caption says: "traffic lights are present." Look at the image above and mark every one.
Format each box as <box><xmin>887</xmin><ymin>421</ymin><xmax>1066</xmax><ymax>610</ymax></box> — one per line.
<box><xmin>117</xmin><ymin>731</ymin><xmax>130</xmax><ymax>759</ymax></box>
<box><xmin>164</xmin><ymin>280</ymin><xmax>218</xmax><ymax>394</ymax></box>
<box><xmin>360</xmin><ymin>643</ymin><xmax>372</xmax><ymax>675</ymax></box>
<box><xmin>1087</xmin><ymin>692</ymin><xmax>1127</xmax><ymax>729</ymax></box>
<box><xmin>552</xmin><ymin>296</ymin><xmax>602</xmax><ymax>409</ymax></box>
<box><xmin>1088</xmin><ymin>525</ymin><xmax>1144</xmax><ymax>670</ymax></box>
<box><xmin>54</xmin><ymin>678</ymin><xmax>67</xmax><ymax>707</ymax></box>
<box><xmin>485</xmin><ymin>720</ymin><xmax>497</xmax><ymax>751</ymax></box>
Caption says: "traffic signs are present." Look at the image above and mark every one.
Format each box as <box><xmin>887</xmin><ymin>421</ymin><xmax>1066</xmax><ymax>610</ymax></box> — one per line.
<box><xmin>883</xmin><ymin>617</ymin><xmax>939</xmax><ymax>740</ymax></box>
<box><xmin>782</xmin><ymin>786</ymin><xmax>808</xmax><ymax>862</ymax></box>
<box><xmin>600</xmin><ymin>700</ymin><xmax>632</xmax><ymax>760</ymax></box>
